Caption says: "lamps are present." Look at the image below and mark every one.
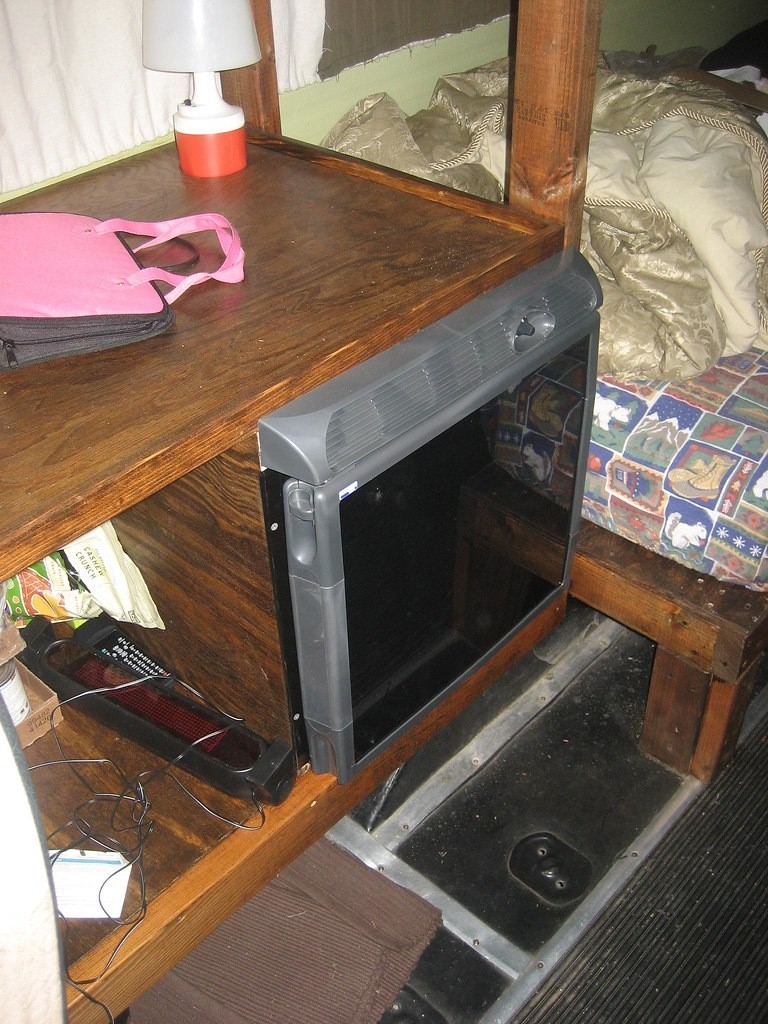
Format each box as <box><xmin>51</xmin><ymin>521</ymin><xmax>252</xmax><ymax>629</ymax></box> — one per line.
<box><xmin>142</xmin><ymin>0</ymin><xmax>262</xmax><ymax>178</ymax></box>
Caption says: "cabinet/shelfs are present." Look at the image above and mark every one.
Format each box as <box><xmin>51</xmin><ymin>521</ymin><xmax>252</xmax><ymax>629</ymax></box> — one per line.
<box><xmin>0</xmin><ymin>0</ymin><xmax>603</xmax><ymax>1022</ymax></box>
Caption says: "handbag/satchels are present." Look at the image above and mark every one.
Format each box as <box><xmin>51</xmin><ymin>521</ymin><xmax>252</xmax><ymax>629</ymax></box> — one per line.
<box><xmin>1</xmin><ymin>211</ymin><xmax>245</xmax><ymax>367</ymax></box>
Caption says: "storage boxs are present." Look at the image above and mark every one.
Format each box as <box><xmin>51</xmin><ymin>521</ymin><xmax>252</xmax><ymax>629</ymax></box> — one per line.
<box><xmin>0</xmin><ymin>610</ymin><xmax>63</xmax><ymax>749</ymax></box>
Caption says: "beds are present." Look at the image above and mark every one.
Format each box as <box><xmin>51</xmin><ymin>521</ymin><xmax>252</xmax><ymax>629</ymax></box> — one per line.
<box><xmin>320</xmin><ymin>0</ymin><xmax>768</xmax><ymax>782</ymax></box>
